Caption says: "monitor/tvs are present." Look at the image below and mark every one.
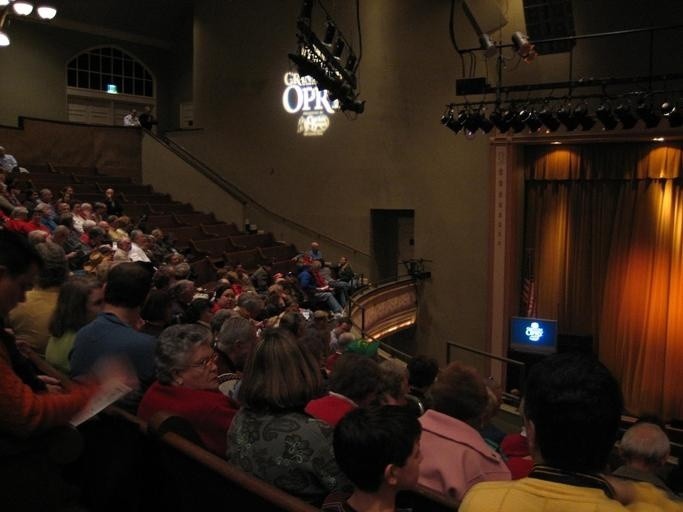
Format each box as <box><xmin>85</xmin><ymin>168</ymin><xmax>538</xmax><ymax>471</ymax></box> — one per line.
<box><xmin>510</xmin><ymin>316</ymin><xmax>559</xmax><ymax>355</ymax></box>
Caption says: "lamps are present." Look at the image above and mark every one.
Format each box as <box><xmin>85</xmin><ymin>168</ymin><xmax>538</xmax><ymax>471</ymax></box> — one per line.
<box><xmin>437</xmin><ymin>90</ymin><xmax>682</xmax><ymax>136</ymax></box>
<box><xmin>285</xmin><ymin>6</ymin><xmax>361</xmax><ymax>115</ymax></box>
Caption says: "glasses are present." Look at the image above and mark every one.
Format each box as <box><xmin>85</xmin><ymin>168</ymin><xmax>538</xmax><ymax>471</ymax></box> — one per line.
<box><xmin>185</xmin><ymin>353</ymin><xmax>218</xmax><ymax>368</ymax></box>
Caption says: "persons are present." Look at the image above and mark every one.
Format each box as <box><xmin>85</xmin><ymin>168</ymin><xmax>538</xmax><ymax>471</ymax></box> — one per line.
<box><xmin>138</xmin><ymin>107</ymin><xmax>157</xmax><ymax>131</ymax></box>
<box><xmin>318</xmin><ymin>403</ymin><xmax>426</xmax><ymax>512</ymax></box>
<box><xmin>123</xmin><ymin>108</ymin><xmax>140</xmax><ymax>127</ymax></box>
<box><xmin>605</xmin><ymin>422</ymin><xmax>675</xmax><ymax>506</ymax></box>
<box><xmin>224</xmin><ymin>331</ymin><xmax>338</xmax><ymax>507</ymax></box>
<box><xmin>414</xmin><ymin>360</ymin><xmax>512</xmax><ymax>499</ymax></box>
<box><xmin>0</xmin><ymin>142</ymin><xmax>542</xmax><ymax>480</ymax></box>
<box><xmin>457</xmin><ymin>348</ymin><xmax>682</xmax><ymax>512</ymax></box>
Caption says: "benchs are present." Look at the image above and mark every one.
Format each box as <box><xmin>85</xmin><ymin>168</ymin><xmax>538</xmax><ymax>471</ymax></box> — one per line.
<box><xmin>0</xmin><ymin>333</ymin><xmax>682</xmax><ymax>512</ymax></box>
<box><xmin>18</xmin><ymin>163</ymin><xmax>306</xmax><ymax>297</ymax></box>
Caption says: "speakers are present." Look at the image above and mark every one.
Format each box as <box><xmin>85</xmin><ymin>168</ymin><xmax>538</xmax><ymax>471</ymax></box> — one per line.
<box><xmin>522</xmin><ymin>0</ymin><xmax>576</xmax><ymax>55</ymax></box>
